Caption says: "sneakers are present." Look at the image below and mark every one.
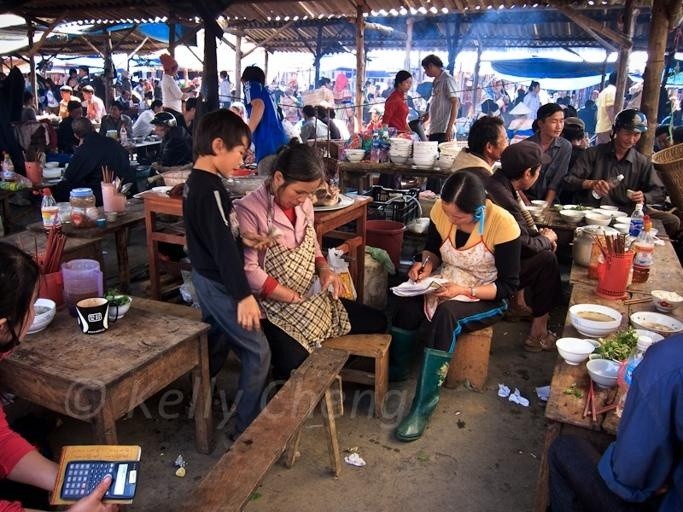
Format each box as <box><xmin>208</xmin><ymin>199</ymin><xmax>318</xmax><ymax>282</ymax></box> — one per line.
<box><xmin>8</xmin><ymin>192</ymin><xmax>32</xmax><ymax>205</ymax></box>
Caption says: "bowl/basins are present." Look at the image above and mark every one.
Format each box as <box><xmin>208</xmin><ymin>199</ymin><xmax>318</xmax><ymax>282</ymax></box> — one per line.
<box><xmin>585</xmin><ymin>358</ymin><xmax>620</xmax><ymax>390</ymax></box>
<box><xmin>651</xmin><ymin>289</ymin><xmax>683</xmax><ymax>313</ymax></box>
<box><xmin>42</xmin><ymin>161</ymin><xmax>68</xmax><ymax>179</ymax></box>
<box><xmin>525</xmin><ymin>198</ymin><xmax>658</xmax><ymax>282</ymax></box>
<box><xmin>555</xmin><ymin>336</ymin><xmax>593</xmax><ymax>365</ymax></box>
<box><xmin>132</xmin><ymin>137</ymin><xmax>145</xmax><ymax>143</ymax></box>
<box><xmin>630</xmin><ymin>310</ymin><xmax>683</xmax><ymax>338</ymax></box>
<box><xmin>107</xmin><ymin>294</ymin><xmax>132</xmax><ymax>320</ymax></box>
<box><xmin>567</xmin><ymin>303</ymin><xmax>625</xmax><ymax>340</ymax></box>
<box><xmin>23</xmin><ymin>297</ymin><xmax>56</xmax><ymax>335</ymax></box>
<box><xmin>346</xmin><ymin>135</ymin><xmax>465</xmax><ymax>170</ymax></box>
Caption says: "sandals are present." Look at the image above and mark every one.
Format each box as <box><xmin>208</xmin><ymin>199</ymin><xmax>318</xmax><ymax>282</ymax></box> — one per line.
<box><xmin>506</xmin><ymin>304</ymin><xmax>532</xmax><ymax>322</ymax></box>
<box><xmin>523</xmin><ymin>329</ymin><xmax>557</xmax><ymax>353</ymax></box>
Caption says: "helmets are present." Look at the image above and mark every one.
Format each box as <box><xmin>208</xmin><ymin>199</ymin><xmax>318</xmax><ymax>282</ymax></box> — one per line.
<box><xmin>611</xmin><ymin>108</ymin><xmax>649</xmax><ymax>133</ymax></box>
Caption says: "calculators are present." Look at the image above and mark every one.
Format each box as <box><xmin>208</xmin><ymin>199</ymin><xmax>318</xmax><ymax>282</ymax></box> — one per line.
<box><xmin>59</xmin><ymin>461</ymin><xmax>140</xmax><ymax>500</ymax></box>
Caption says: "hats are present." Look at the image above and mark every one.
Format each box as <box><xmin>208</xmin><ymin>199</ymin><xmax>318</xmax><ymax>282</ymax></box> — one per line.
<box><xmin>563</xmin><ymin>116</ymin><xmax>585</xmax><ymax>129</ymax></box>
<box><xmin>149</xmin><ymin>112</ymin><xmax>176</xmax><ymax>127</ymax></box>
<box><xmin>500</xmin><ymin>140</ymin><xmax>553</xmax><ymax>177</ymax></box>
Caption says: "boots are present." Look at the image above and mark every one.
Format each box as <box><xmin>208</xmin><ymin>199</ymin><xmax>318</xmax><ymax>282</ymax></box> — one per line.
<box><xmin>394</xmin><ymin>346</ymin><xmax>454</xmax><ymax>442</ymax></box>
<box><xmin>384</xmin><ymin>325</ymin><xmax>418</xmax><ymax>382</ymax></box>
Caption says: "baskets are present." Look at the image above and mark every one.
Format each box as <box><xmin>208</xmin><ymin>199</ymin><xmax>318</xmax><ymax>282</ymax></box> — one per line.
<box><xmin>650</xmin><ymin>142</ymin><xmax>682</xmax><ymax>207</ymax></box>
<box><xmin>347</xmin><ymin>184</ymin><xmax>420</xmax><ymax>231</ymax></box>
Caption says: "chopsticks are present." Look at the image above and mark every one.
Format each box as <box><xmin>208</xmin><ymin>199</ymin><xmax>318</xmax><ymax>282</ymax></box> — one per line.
<box><xmin>587</xmin><ymin>403</ymin><xmax>618</xmax><ymax>417</ymax></box>
<box><xmin>100</xmin><ymin>165</ymin><xmax>116</xmax><ymax>184</ymax></box>
<box><xmin>622</xmin><ymin>293</ymin><xmax>656</xmax><ymax>305</ymax></box>
<box><xmin>20</xmin><ymin>146</ymin><xmax>42</xmax><ymax>161</ymax></box>
<box><xmin>33</xmin><ymin>208</ymin><xmax>68</xmax><ymax>275</ymax></box>
<box><xmin>581</xmin><ymin>378</ymin><xmax>598</xmax><ymax>423</ymax></box>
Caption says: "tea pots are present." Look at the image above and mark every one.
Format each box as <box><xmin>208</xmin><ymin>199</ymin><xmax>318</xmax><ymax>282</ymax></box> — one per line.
<box><xmin>61</xmin><ymin>259</ymin><xmax>105</xmax><ymax>320</ymax></box>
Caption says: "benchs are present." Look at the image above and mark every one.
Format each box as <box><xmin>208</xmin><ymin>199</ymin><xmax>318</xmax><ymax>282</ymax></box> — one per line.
<box><xmin>0</xmin><ymin>138</ymin><xmax>681</xmax><ymax>509</ymax></box>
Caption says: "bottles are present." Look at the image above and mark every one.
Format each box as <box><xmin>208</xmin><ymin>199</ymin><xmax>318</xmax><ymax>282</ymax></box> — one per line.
<box><xmin>615</xmin><ymin>334</ymin><xmax>653</xmax><ymax>418</ymax></box>
<box><xmin>2</xmin><ymin>153</ymin><xmax>15</xmax><ymax>182</ymax></box>
<box><xmin>67</xmin><ymin>186</ymin><xmax>99</xmax><ymax>226</ymax></box>
<box><xmin>592</xmin><ymin>174</ymin><xmax>626</xmax><ymax>199</ymax></box>
<box><xmin>39</xmin><ymin>187</ymin><xmax>63</xmax><ymax>238</ymax></box>
<box><xmin>106</xmin><ymin>126</ymin><xmax>128</xmax><ymax>146</ymax></box>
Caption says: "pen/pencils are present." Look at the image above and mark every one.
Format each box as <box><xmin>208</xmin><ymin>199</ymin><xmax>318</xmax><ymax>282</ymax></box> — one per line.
<box><xmin>413</xmin><ymin>256</ymin><xmax>431</xmax><ymax>285</ymax></box>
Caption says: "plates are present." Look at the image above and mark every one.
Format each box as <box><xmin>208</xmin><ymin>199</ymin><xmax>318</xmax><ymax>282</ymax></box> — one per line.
<box><xmin>630</xmin><ymin>328</ymin><xmax>665</xmax><ymax>348</ymax></box>
<box><xmin>310</xmin><ymin>192</ymin><xmax>354</xmax><ymax>211</ymax></box>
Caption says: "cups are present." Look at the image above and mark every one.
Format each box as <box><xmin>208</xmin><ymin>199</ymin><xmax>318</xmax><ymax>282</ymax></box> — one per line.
<box><xmin>105</xmin><ymin>211</ymin><xmax>116</xmax><ymax>222</ymax></box>
<box><xmin>96</xmin><ymin>218</ymin><xmax>108</xmax><ymax>228</ymax></box>
<box><xmin>627</xmin><ymin>189</ymin><xmax>633</xmax><ymax>198</ymax></box>
<box><xmin>75</xmin><ymin>296</ymin><xmax>118</xmax><ymax>335</ymax></box>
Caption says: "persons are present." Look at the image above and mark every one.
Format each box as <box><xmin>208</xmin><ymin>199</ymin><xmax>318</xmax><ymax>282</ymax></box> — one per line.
<box><xmin>546</xmin><ymin>329</ymin><xmax>682</xmax><ymax>511</ymax></box>
<box><xmin>1</xmin><ymin>240</ymin><xmax>117</xmax><ymax>511</ymax></box>
